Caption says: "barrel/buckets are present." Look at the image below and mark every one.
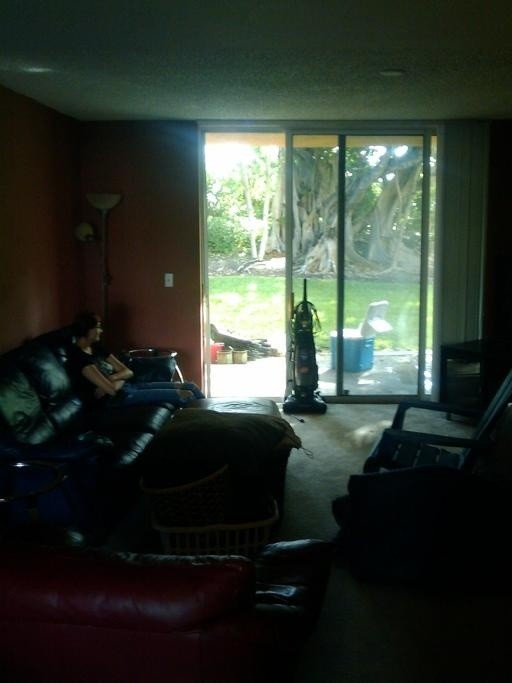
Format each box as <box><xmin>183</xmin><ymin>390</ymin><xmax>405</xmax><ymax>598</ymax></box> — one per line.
<box><xmin>211</xmin><ymin>345</ymin><xmax>224</xmax><ymax>362</ymax></box>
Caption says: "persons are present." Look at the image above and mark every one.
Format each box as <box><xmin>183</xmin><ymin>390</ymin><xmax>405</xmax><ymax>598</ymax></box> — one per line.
<box><xmin>69</xmin><ymin>307</ymin><xmax>207</xmax><ymax>410</ymax></box>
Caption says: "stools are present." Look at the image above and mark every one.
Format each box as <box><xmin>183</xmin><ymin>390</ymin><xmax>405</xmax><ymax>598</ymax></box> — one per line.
<box><xmin>147</xmin><ymin>394</ymin><xmax>289</xmax><ymax>528</ymax></box>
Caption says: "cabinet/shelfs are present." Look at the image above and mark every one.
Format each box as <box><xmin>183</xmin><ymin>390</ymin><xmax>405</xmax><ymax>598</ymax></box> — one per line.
<box><xmin>442</xmin><ymin>338</ymin><xmax>508</xmax><ymax>420</ymax></box>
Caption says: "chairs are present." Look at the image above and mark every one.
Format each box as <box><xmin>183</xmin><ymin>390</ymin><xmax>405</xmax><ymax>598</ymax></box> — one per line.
<box><xmin>331</xmin><ymin>348</ymin><xmax>512</xmax><ymax>586</ymax></box>
<box><xmin>3</xmin><ymin>519</ymin><xmax>337</xmax><ymax>681</ymax></box>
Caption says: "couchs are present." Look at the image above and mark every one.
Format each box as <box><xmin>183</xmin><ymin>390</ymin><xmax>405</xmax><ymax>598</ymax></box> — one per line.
<box><xmin>0</xmin><ymin>320</ymin><xmax>186</xmax><ymax>506</ymax></box>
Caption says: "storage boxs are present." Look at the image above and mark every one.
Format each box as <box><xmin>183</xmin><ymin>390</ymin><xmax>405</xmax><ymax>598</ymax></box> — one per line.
<box><xmin>329</xmin><ymin>297</ymin><xmax>392</xmax><ymax>374</ymax></box>
